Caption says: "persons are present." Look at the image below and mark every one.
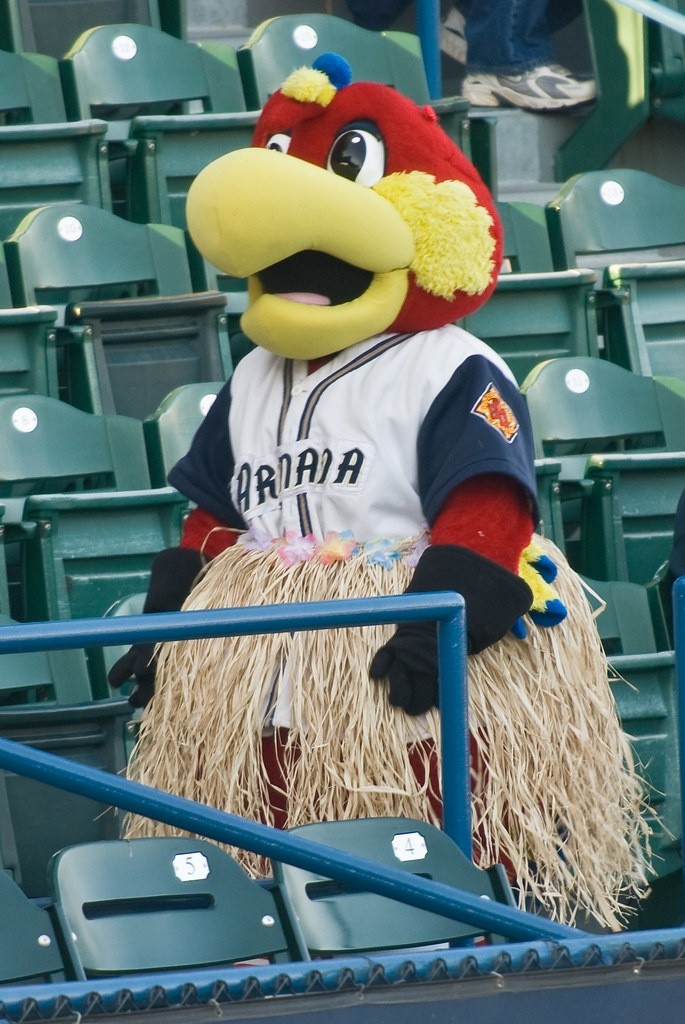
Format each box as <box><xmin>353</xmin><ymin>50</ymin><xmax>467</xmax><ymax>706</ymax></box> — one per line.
<box><xmin>438</xmin><ymin>0</ymin><xmax>596</xmax><ymax>109</ymax></box>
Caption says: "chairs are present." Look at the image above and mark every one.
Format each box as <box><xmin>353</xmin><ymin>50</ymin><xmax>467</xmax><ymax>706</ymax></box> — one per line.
<box><xmin>0</xmin><ymin>0</ymin><xmax>685</xmax><ymax>989</ymax></box>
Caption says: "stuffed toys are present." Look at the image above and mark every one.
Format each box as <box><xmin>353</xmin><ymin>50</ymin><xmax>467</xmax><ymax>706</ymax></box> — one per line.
<box><xmin>108</xmin><ymin>52</ymin><xmax>676</xmax><ymax>931</ymax></box>
